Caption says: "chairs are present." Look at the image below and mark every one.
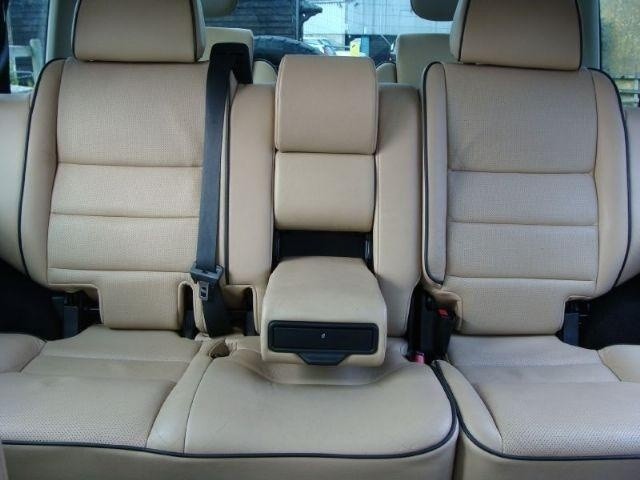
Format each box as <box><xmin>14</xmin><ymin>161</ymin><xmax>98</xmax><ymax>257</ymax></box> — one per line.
<box><xmin>0</xmin><ymin>1</ymin><xmax>640</xmax><ymax>480</ymax></box>
